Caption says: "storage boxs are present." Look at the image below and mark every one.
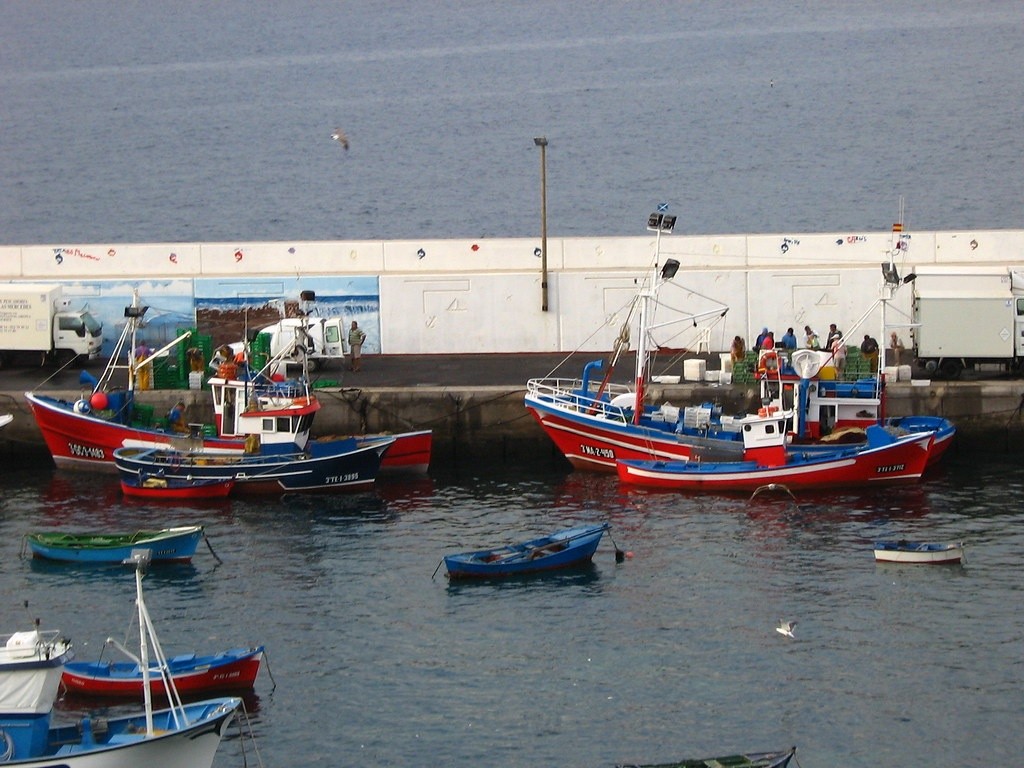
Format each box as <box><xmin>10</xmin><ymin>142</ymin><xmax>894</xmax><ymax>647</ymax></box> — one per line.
<box><xmin>648</xmin><ymin>400</ymin><xmax>742</xmax><ymax>433</ymax></box>
<box><xmin>6</xmin><ymin>631</ymin><xmax>39</xmax><ymax>657</ymax></box>
<box><xmin>651</xmin><ymin>348</ymin><xmax>911</xmax><ymax>399</ymax></box>
<box><xmin>126</xmin><ymin>404</ymin><xmax>217</xmax><ymax>439</ymax></box>
<box><xmin>132</xmin><ymin>327</ymin><xmax>272</xmax><ymax>391</ymax></box>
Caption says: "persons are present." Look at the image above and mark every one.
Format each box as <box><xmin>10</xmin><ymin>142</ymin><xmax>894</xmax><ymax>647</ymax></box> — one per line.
<box><xmin>829</xmin><ymin>333</ymin><xmax>847</xmax><ymax>380</ymax></box>
<box><xmin>348</xmin><ymin>321</ymin><xmax>366</xmax><ymax>373</ymax></box>
<box><xmin>220</xmin><ymin>344</ymin><xmax>235</xmax><ymax>363</ymax></box>
<box><xmin>826</xmin><ymin>324</ymin><xmax>843</xmax><ymax>349</ymax></box>
<box><xmin>186</xmin><ymin>348</ymin><xmax>204</xmax><ymax>373</ymax></box>
<box><xmin>889</xmin><ymin>332</ymin><xmax>905</xmax><ymax>366</ymax></box>
<box><xmin>802</xmin><ymin>326</ymin><xmax>820</xmax><ymax>349</ymax></box>
<box><xmin>170</xmin><ymin>401</ymin><xmax>190</xmax><ymax>433</ymax></box>
<box><xmin>861</xmin><ymin>335</ymin><xmax>880</xmax><ymax>373</ymax></box>
<box><xmin>134</xmin><ymin>338</ymin><xmax>156</xmax><ymax>391</ymax></box>
<box><xmin>782</xmin><ymin>328</ymin><xmax>797</xmax><ymax>351</ymax></box>
<box><xmin>755</xmin><ymin>328</ymin><xmax>775</xmax><ymax>350</ymax></box>
<box><xmin>730</xmin><ymin>335</ymin><xmax>747</xmax><ymax>364</ymax></box>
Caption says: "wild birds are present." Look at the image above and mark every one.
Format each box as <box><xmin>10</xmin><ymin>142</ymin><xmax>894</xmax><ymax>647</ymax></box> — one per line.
<box><xmin>775</xmin><ymin>617</ymin><xmax>797</xmax><ymax>637</ymax></box>
<box><xmin>331</xmin><ymin>126</ymin><xmax>350</xmax><ymax>151</ymax></box>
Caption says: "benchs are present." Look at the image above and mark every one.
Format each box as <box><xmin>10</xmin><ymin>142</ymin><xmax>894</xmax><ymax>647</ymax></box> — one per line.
<box><xmin>525</xmin><ymin>545</ymin><xmax>556</xmax><ymax>556</ymax></box>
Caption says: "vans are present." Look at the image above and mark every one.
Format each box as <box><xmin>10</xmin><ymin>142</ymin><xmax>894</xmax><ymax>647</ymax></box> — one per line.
<box><xmin>228</xmin><ymin>318</ymin><xmax>351</xmax><ymax>374</ymax></box>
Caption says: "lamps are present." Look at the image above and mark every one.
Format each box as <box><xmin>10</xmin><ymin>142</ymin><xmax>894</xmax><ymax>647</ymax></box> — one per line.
<box><xmin>782</xmin><ymin>240</ymin><xmax>788</xmax><ymax>253</ymax></box>
<box><xmin>124</xmin><ymin>305</ymin><xmax>148</xmax><ymax>318</ymax></box>
<box><xmin>647</xmin><ymin>212</ymin><xmax>677</xmax><ymax>231</ymax></box>
<box><xmin>170</xmin><ymin>253</ymin><xmax>178</xmax><ymax>264</ymax></box>
<box><xmin>534</xmin><ymin>247</ymin><xmax>542</xmax><ymax>257</ymax></box>
<box><xmin>970</xmin><ymin>239</ymin><xmax>978</xmax><ymax>251</ymax></box>
<box><xmin>417</xmin><ymin>248</ymin><xmax>425</xmax><ymax>259</ymax></box>
<box><xmin>56</xmin><ymin>254</ymin><xmax>63</xmax><ymax>264</ymax></box>
<box><xmin>235</xmin><ymin>251</ymin><xmax>242</xmax><ymax>263</ymax></box>
<box><xmin>301</xmin><ymin>291</ymin><xmax>316</xmax><ymax>302</ymax></box>
<box><xmin>882</xmin><ymin>263</ymin><xmax>917</xmax><ymax>285</ymax></box>
<box><xmin>658</xmin><ymin>258</ymin><xmax>681</xmax><ymax>279</ymax></box>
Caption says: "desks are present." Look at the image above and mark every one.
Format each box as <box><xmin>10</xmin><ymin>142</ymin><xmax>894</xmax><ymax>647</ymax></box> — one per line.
<box><xmin>171</xmin><ymin>655</ymin><xmax>196</xmax><ymax>673</ymax></box>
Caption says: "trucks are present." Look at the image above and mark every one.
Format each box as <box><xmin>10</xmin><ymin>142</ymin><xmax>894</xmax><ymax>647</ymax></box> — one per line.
<box><xmin>912</xmin><ymin>284</ymin><xmax>1024</xmax><ymax>379</ymax></box>
<box><xmin>0</xmin><ymin>283</ymin><xmax>103</xmax><ymax>374</ymax></box>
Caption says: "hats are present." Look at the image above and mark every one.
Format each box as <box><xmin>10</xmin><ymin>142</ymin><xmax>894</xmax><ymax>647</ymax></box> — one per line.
<box><xmin>890</xmin><ymin>331</ymin><xmax>896</xmax><ymax>337</ymax></box>
<box><xmin>829</xmin><ymin>334</ymin><xmax>840</xmax><ymax>339</ymax></box>
<box><xmin>788</xmin><ymin>328</ymin><xmax>793</xmax><ymax>334</ymax></box>
<box><xmin>763</xmin><ymin>328</ymin><xmax>768</xmax><ymax>333</ymax></box>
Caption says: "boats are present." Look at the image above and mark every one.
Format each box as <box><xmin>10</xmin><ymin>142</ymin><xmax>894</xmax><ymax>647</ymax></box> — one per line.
<box><xmin>22</xmin><ymin>288</ymin><xmax>433</xmax><ymax>501</ymax></box>
<box><xmin>871</xmin><ymin>539</ymin><xmax>965</xmax><ymax>564</ymax></box>
<box><xmin>524</xmin><ymin>194</ymin><xmax>959</xmax><ymax>494</ymax></box>
<box><xmin>0</xmin><ymin>555</ymin><xmax>266</xmax><ymax>768</ymax></box>
<box><xmin>26</xmin><ymin>524</ymin><xmax>206</xmax><ymax>567</ymax></box>
<box><xmin>443</xmin><ymin>521</ymin><xmax>612</xmax><ymax>580</ymax></box>
<box><xmin>612</xmin><ymin>744</ymin><xmax>798</xmax><ymax>768</ymax></box>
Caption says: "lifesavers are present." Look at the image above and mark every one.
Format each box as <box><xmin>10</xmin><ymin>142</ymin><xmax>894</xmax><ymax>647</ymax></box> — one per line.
<box><xmin>758</xmin><ymin>406</ymin><xmax>779</xmax><ymax>413</ymax></box>
<box><xmin>293</xmin><ymin>396</ymin><xmax>317</xmax><ymax>406</ymax></box>
<box><xmin>758</xmin><ymin>412</ymin><xmax>772</xmax><ymax>417</ymax></box>
<box><xmin>760</xmin><ymin>352</ymin><xmax>782</xmax><ymax>375</ymax></box>
<box><xmin>218</xmin><ymin>363</ymin><xmax>238</xmax><ymax>380</ymax></box>
